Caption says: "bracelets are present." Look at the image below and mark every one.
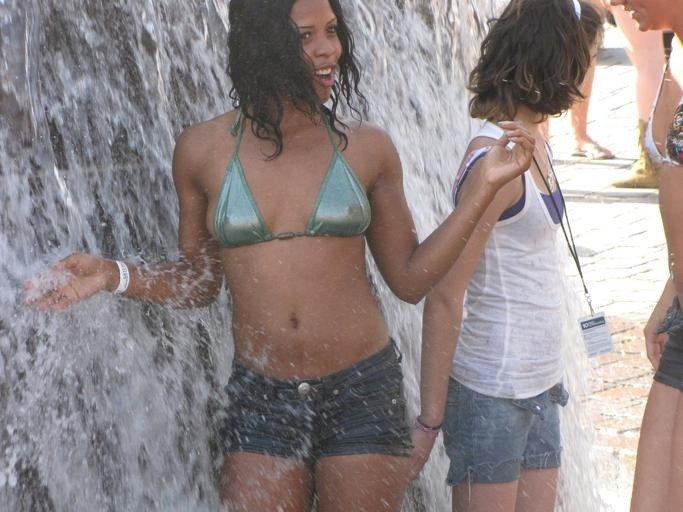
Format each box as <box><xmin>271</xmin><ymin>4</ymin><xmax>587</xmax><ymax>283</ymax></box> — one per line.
<box><xmin>111</xmin><ymin>260</ymin><xmax>131</xmax><ymax>296</ymax></box>
<box><xmin>414</xmin><ymin>416</ymin><xmax>443</xmax><ymax>436</ymax></box>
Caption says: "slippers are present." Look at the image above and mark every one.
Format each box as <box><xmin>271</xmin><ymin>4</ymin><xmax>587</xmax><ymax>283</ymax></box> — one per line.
<box><xmin>572</xmin><ymin>141</ymin><xmax>614</xmax><ymax>160</ymax></box>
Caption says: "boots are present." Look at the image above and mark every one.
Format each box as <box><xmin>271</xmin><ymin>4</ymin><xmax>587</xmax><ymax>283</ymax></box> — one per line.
<box><xmin>612</xmin><ymin>117</ymin><xmax>660</xmax><ymax>188</ymax></box>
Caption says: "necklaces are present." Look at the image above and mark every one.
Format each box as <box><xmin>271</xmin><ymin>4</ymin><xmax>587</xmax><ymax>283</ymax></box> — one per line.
<box><xmin>534</xmin><ymin>142</ymin><xmax>555</xmax><ymax>186</ymax></box>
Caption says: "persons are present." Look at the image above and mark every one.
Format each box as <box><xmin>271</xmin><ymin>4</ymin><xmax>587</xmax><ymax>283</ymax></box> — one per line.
<box><xmin>598</xmin><ymin>0</ymin><xmax>675</xmax><ymax>189</ymax></box>
<box><xmin>534</xmin><ymin>0</ymin><xmax>616</xmax><ymax>162</ymax></box>
<box><xmin>409</xmin><ymin>1</ymin><xmax>607</xmax><ymax>510</ymax></box>
<box><xmin>605</xmin><ymin>0</ymin><xmax>681</xmax><ymax>512</ymax></box>
<box><xmin>21</xmin><ymin>0</ymin><xmax>536</xmax><ymax>512</ymax></box>
